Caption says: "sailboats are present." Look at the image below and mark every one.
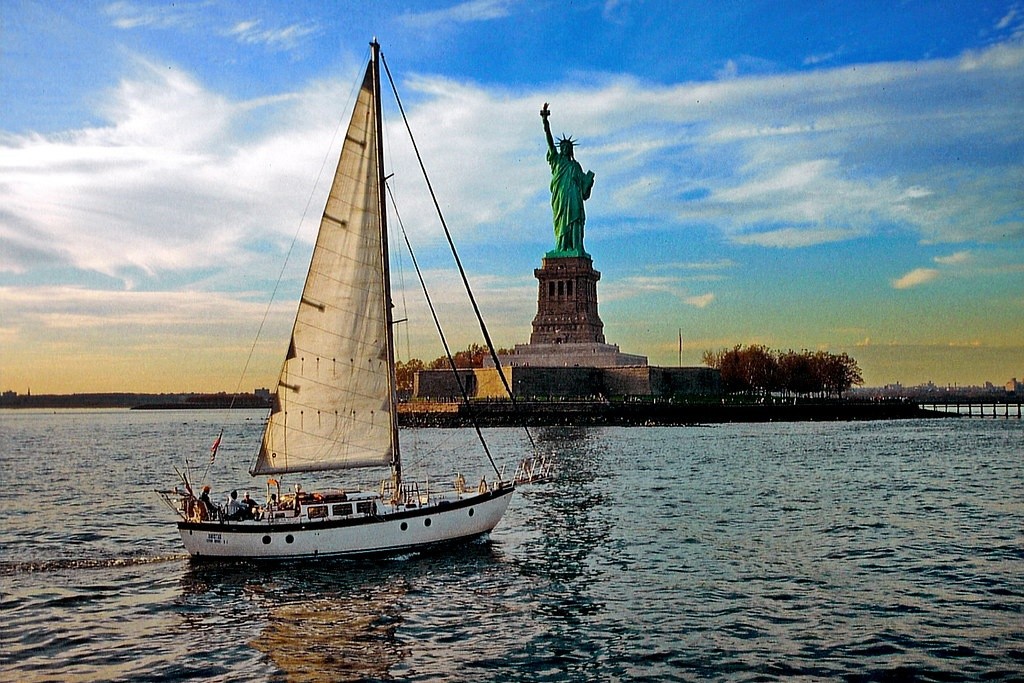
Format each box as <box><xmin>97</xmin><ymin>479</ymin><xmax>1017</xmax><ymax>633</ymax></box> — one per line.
<box><xmin>153</xmin><ymin>36</ymin><xmax>552</xmax><ymax>561</ymax></box>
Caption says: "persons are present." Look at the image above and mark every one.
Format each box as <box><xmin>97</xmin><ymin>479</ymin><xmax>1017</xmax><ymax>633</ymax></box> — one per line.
<box><xmin>199</xmin><ymin>486</ymin><xmax>218</xmax><ymax>514</ymax></box>
<box><xmin>227</xmin><ymin>489</ymin><xmax>259</xmax><ymax>520</ymax></box>
<box><xmin>543</xmin><ymin>120</ymin><xmax>594</xmax><ymax>252</ymax></box>
<box><xmin>397</xmin><ymin>395</ymin><xmax>796</xmax><ymax>405</ymax></box>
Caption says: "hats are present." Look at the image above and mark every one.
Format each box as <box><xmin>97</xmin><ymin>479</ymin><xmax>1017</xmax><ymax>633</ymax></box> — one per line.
<box><xmin>245</xmin><ymin>492</ymin><xmax>250</xmax><ymax>495</ymax></box>
<box><xmin>204</xmin><ymin>486</ymin><xmax>211</xmax><ymax>491</ymax></box>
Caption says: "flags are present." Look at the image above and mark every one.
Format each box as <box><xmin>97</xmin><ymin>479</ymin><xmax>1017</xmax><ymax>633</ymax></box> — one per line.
<box><xmin>210</xmin><ymin>437</ymin><xmax>221</xmax><ymax>464</ymax></box>
